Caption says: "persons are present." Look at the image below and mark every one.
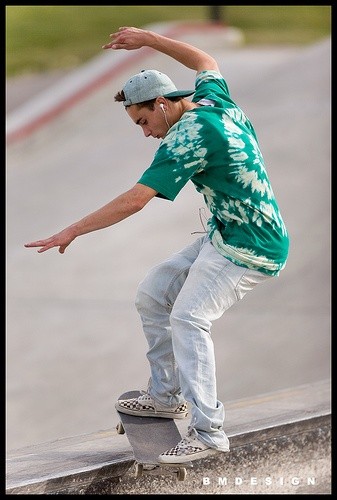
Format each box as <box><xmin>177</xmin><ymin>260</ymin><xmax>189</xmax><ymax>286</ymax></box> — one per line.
<box><xmin>23</xmin><ymin>26</ymin><xmax>289</xmax><ymax>464</ymax></box>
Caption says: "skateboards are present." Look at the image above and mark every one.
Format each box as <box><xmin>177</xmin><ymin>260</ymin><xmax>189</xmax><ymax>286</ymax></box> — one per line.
<box><xmin>115</xmin><ymin>390</ymin><xmax>187</xmax><ymax>482</ymax></box>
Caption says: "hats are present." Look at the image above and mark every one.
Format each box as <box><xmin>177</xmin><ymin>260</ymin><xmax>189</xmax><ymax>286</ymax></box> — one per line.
<box><xmin>121</xmin><ymin>69</ymin><xmax>196</xmax><ymax>108</ymax></box>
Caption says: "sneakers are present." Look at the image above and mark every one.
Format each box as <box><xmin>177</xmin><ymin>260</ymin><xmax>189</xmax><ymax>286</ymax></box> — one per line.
<box><xmin>156</xmin><ymin>417</ymin><xmax>222</xmax><ymax>464</ymax></box>
<box><xmin>115</xmin><ymin>385</ymin><xmax>189</xmax><ymax>420</ymax></box>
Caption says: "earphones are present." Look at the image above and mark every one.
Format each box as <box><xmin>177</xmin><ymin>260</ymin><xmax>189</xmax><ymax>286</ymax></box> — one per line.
<box><xmin>159</xmin><ymin>103</ymin><xmax>166</xmax><ymax>111</ymax></box>
